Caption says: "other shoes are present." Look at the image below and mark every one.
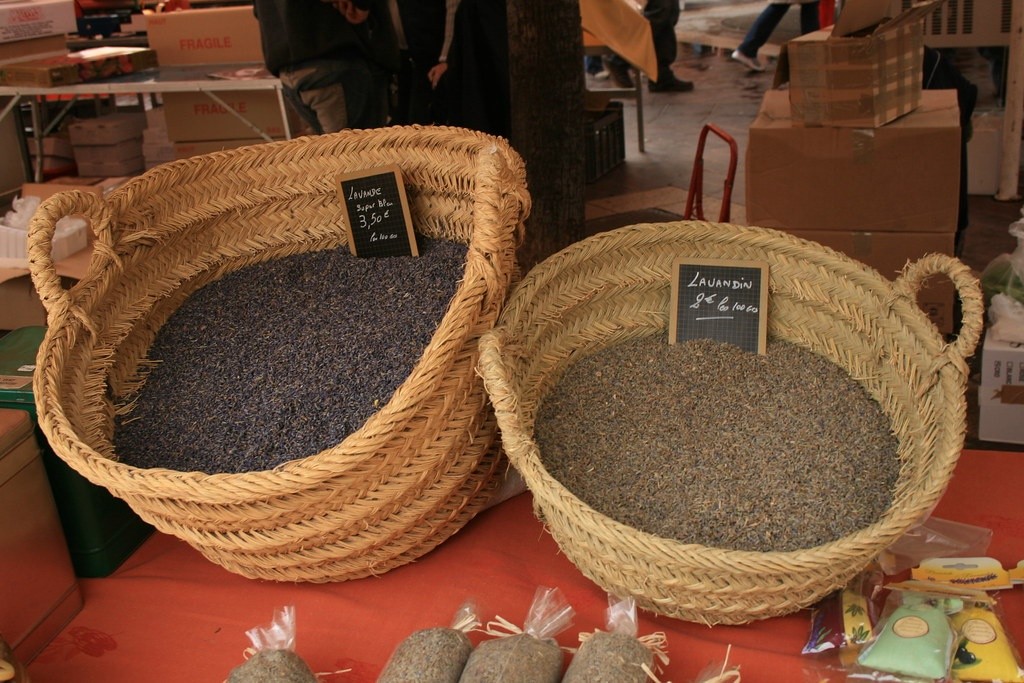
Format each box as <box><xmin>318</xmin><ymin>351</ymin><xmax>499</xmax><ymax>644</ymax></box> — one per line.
<box><xmin>732</xmin><ymin>49</ymin><xmax>764</xmax><ymax>71</ymax></box>
<box><xmin>585</xmin><ymin>69</ymin><xmax>610</xmax><ymax>80</ymax></box>
<box><xmin>649</xmin><ymin>72</ymin><xmax>693</xmax><ymax>92</ymax></box>
<box><xmin>604</xmin><ymin>58</ymin><xmax>633</xmax><ymax>88</ymax></box>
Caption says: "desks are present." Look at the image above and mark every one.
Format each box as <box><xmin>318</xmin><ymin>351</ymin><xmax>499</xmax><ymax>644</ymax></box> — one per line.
<box><xmin>13</xmin><ymin>450</ymin><xmax>1024</xmax><ymax>683</ymax></box>
<box><xmin>0</xmin><ymin>63</ymin><xmax>293</xmax><ymax>184</ymax></box>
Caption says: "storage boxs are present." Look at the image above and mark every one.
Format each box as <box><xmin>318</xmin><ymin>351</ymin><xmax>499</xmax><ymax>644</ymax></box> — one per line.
<box><xmin>0</xmin><ymin>183</ymin><xmax>104</xmax><ymax>331</ymax></box>
<box><xmin>588</xmin><ymin>100</ymin><xmax>626</xmax><ymax>182</ymax></box>
<box><xmin>28</xmin><ymin>112</ymin><xmax>148</xmax><ymax>190</ymax></box>
<box><xmin>977</xmin><ymin>326</ymin><xmax>1024</xmax><ymax>446</ymax></box>
<box><xmin>744</xmin><ymin>0</ymin><xmax>962</xmax><ymax>334</ymax></box>
<box><xmin>145</xmin><ymin>5</ymin><xmax>301</xmax><ymax>164</ymax></box>
<box><xmin>0</xmin><ymin>0</ymin><xmax>157</xmax><ymax>88</ymax></box>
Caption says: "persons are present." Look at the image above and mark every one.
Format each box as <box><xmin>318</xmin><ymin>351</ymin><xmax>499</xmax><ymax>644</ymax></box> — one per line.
<box><xmin>731</xmin><ymin>0</ymin><xmax>822</xmax><ymax>72</ymax></box>
<box><xmin>253</xmin><ymin>0</ymin><xmax>510</xmax><ymax>137</ymax></box>
<box><xmin>581</xmin><ymin>0</ymin><xmax>693</xmax><ymax>91</ymax></box>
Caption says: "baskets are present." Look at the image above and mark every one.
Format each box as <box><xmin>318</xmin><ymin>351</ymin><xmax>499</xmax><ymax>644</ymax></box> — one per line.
<box><xmin>478</xmin><ymin>220</ymin><xmax>984</xmax><ymax>626</ymax></box>
<box><xmin>26</xmin><ymin>125</ymin><xmax>530</xmax><ymax>584</ymax></box>
